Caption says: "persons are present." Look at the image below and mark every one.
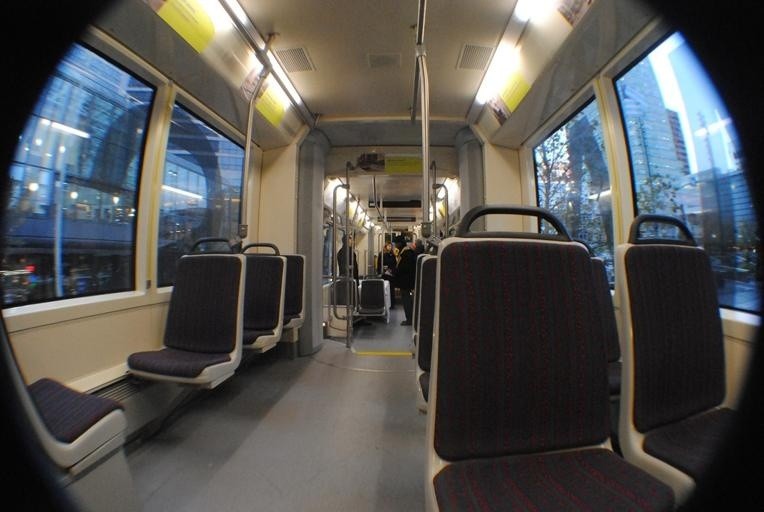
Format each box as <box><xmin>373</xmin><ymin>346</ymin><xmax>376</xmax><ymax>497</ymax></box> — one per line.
<box><xmin>376</xmin><ymin>241</ymin><xmax>398</xmax><ymax>309</ymax></box>
<box><xmin>336</xmin><ymin>235</ymin><xmax>371</xmax><ymax>326</ymax></box>
<box><xmin>391</xmin><ymin>239</ymin><xmax>424</xmax><ymax>326</ymax></box>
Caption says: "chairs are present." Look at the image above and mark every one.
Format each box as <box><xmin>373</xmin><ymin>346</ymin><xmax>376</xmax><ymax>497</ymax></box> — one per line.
<box><xmin>426</xmin><ymin>204</ymin><xmax>677</xmax><ymax>512</ymax></box>
<box><xmin>274</xmin><ymin>252</ymin><xmax>308</xmax><ymax>347</ymax></box>
<box><xmin>411</xmin><ymin>240</ymin><xmax>438</xmax><ymax>405</ymax></box>
<box><xmin>0</xmin><ymin>289</ymin><xmax>127</xmax><ymax>481</ymax></box>
<box><xmin>234</xmin><ymin>242</ymin><xmax>285</xmax><ymax>358</ymax></box>
<box><xmin>328</xmin><ymin>273</ymin><xmax>389</xmax><ymax>324</ymax></box>
<box><xmin>611</xmin><ymin>212</ymin><xmax>739</xmax><ymax>500</ymax></box>
<box><xmin>122</xmin><ymin>237</ymin><xmax>247</xmax><ymax>392</ymax></box>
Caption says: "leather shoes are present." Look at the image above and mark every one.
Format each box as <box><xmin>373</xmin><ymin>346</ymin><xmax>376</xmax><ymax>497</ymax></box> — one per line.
<box><xmin>357</xmin><ymin>321</ymin><xmax>371</xmax><ymax>325</ymax></box>
<box><xmin>401</xmin><ymin>322</ymin><xmax>411</xmax><ymax>325</ymax></box>
